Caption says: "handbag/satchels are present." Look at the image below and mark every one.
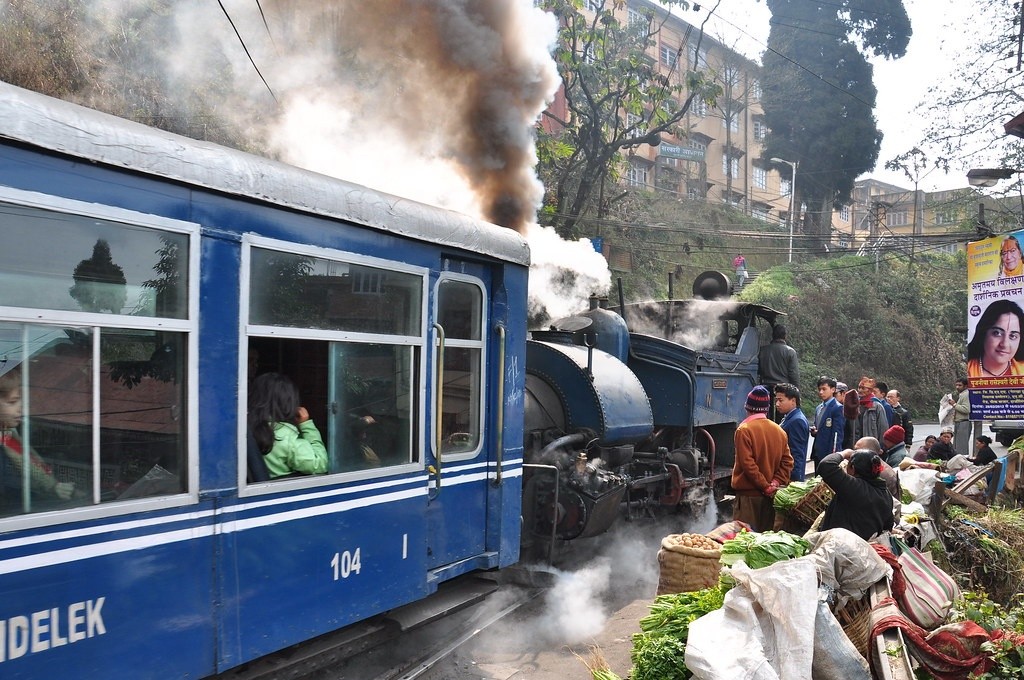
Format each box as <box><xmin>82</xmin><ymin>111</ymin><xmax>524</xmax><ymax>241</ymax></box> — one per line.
<box><xmin>890</xmin><ymin>538</ymin><xmax>966</xmax><ymax>632</ymax></box>
<box><xmin>743</xmin><ymin>270</ymin><xmax>748</xmax><ymax>279</ymax></box>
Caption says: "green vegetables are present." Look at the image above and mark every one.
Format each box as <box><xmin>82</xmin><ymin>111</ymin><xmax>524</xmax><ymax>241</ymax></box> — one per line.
<box><xmin>926</xmin><ymin>458</ymin><xmax>943</xmax><ymax>464</ymax></box>
<box><xmin>720</xmin><ymin>528</ymin><xmax>814</xmax><ymax>570</ymax></box>
<box><xmin>773</xmin><ymin>475</ymin><xmax>821</xmax><ymax>515</ymax></box>
<box><xmin>902</xmin><ymin>489</ymin><xmax>912</xmax><ymax>504</ymax></box>
<box><xmin>581</xmin><ymin>586</ymin><xmax>724</xmax><ymax>679</ymax></box>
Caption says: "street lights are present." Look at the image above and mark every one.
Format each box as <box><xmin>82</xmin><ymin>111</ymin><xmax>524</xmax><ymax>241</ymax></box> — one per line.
<box><xmin>769</xmin><ymin>157</ymin><xmax>796</xmax><ymax>262</ymax></box>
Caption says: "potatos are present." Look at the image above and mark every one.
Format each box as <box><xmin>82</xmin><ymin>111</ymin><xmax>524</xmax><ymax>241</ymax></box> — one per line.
<box><xmin>676</xmin><ymin>533</ymin><xmax>720</xmax><ymax>551</ymax></box>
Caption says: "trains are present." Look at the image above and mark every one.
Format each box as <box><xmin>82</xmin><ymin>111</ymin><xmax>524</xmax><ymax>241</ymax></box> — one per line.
<box><xmin>0</xmin><ymin>79</ymin><xmax>786</xmax><ymax>680</ymax></box>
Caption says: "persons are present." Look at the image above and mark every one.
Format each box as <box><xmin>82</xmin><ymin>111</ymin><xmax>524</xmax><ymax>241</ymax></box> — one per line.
<box><xmin>731</xmin><ymin>385</ymin><xmax>794</xmax><ymax>534</ymax></box>
<box><xmin>997</xmin><ymin>236</ymin><xmax>1024</xmax><ymax>278</ymax></box>
<box><xmin>812</xmin><ymin>375</ymin><xmax>997</xmax><ymax>543</ymax></box>
<box><xmin>736</xmin><ymin>253</ymin><xmax>747</xmax><ymax>286</ymax></box>
<box><xmin>248</xmin><ymin>371</ymin><xmax>329</xmax><ymax>479</ymax></box>
<box><xmin>774</xmin><ymin>383</ymin><xmax>809</xmax><ymax>482</ymax></box>
<box><xmin>0</xmin><ymin>369</ymin><xmax>75</xmax><ymax>503</ymax></box>
<box><xmin>967</xmin><ymin>299</ymin><xmax>1024</xmax><ymax>375</ymax></box>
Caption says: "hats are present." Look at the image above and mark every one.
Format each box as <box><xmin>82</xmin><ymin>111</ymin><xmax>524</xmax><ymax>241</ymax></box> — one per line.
<box><xmin>885</xmin><ymin>425</ymin><xmax>906</xmax><ymax>444</ymax></box>
<box><xmin>858</xmin><ymin>377</ymin><xmax>875</xmax><ymax>389</ymax></box>
<box><xmin>833</xmin><ymin>383</ymin><xmax>849</xmax><ymax>392</ymax></box>
<box><xmin>744</xmin><ymin>386</ymin><xmax>771</xmax><ymax>412</ymax></box>
<box><xmin>939</xmin><ymin>427</ymin><xmax>955</xmax><ymax>437</ymax></box>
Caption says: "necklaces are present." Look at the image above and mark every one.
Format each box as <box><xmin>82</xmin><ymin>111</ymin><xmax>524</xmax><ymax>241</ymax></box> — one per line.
<box><xmin>982</xmin><ymin>367</ymin><xmax>1010</xmax><ymax>376</ymax></box>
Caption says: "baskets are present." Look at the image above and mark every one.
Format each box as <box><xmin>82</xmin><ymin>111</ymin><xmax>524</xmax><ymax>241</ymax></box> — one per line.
<box><xmin>830</xmin><ymin>597</ymin><xmax>874</xmax><ymax>657</ymax></box>
<box><xmin>788</xmin><ymin>482</ymin><xmax>835</xmax><ymax>528</ymax></box>
